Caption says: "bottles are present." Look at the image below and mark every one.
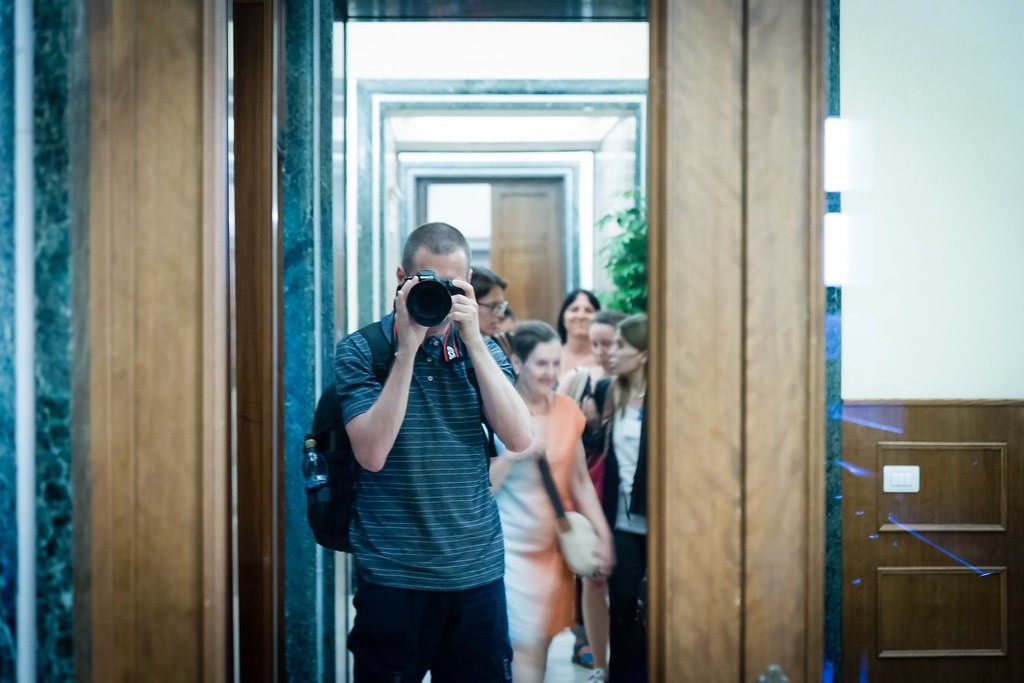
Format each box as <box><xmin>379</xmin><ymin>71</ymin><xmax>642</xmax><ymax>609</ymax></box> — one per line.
<box><xmin>303</xmin><ymin>438</ymin><xmax>328</xmax><ymax>488</ymax></box>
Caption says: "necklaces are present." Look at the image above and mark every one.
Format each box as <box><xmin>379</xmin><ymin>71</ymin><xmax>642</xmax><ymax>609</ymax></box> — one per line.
<box><xmin>632</xmin><ymin>394</ymin><xmax>644</xmax><ymax>399</ymax></box>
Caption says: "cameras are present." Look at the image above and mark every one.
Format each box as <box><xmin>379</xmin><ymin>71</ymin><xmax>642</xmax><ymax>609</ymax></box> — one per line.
<box><xmin>404</xmin><ymin>270</ymin><xmax>464</xmax><ymax>327</ymax></box>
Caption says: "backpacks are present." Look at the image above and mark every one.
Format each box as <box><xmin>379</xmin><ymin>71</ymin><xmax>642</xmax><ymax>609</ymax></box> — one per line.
<box><xmin>302</xmin><ymin>322</ymin><xmax>397</xmax><ymax>553</ymax></box>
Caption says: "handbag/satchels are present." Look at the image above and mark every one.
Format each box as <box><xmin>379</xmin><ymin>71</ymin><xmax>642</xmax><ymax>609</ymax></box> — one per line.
<box><xmin>533</xmin><ymin>450</ymin><xmax>607</xmax><ymax>580</ymax></box>
<box><xmin>585</xmin><ymin>450</ymin><xmax>605</xmax><ymax>504</ymax></box>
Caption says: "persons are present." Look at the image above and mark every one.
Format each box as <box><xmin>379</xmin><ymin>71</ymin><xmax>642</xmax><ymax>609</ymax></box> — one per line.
<box><xmin>470</xmin><ymin>265</ymin><xmax>614</xmax><ymax>683</ymax></box>
<box><xmin>557</xmin><ymin>289</ymin><xmax>649</xmax><ymax>683</ymax></box>
<box><xmin>336</xmin><ymin>222</ymin><xmax>533</xmax><ymax>683</ymax></box>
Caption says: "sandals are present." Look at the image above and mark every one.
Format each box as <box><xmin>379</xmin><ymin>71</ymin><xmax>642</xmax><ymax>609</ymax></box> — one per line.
<box><xmin>572</xmin><ymin>641</ymin><xmax>594</xmax><ymax>670</ymax></box>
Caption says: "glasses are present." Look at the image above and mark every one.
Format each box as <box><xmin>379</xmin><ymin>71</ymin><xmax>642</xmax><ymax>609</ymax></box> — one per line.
<box><xmin>476</xmin><ymin>301</ymin><xmax>508</xmax><ymax>316</ymax></box>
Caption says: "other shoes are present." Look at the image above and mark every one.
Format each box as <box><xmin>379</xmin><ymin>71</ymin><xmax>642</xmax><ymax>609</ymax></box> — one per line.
<box><xmin>585</xmin><ymin>668</ymin><xmax>609</xmax><ymax>683</ymax></box>
